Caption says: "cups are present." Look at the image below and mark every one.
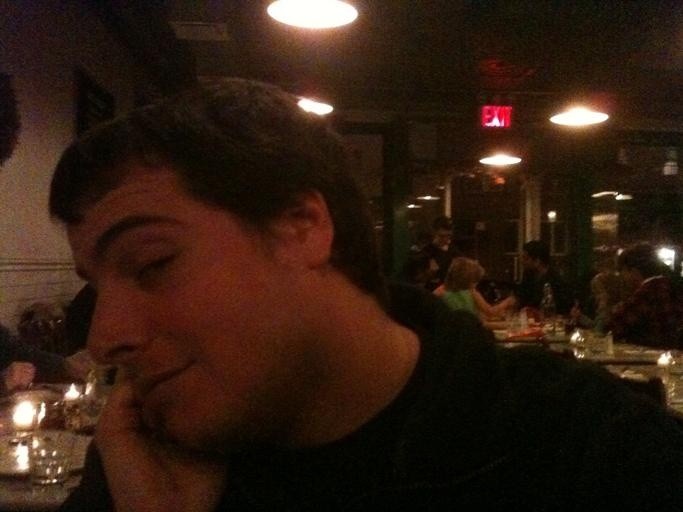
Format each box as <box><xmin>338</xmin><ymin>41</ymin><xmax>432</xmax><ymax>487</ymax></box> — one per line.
<box><xmin>28</xmin><ymin>404</ymin><xmax>78</xmax><ymax>485</ymax></box>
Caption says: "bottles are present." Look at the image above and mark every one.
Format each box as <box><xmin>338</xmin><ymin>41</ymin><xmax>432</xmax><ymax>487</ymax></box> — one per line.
<box><xmin>604</xmin><ymin>331</ymin><xmax>614</xmax><ymax>354</ymax></box>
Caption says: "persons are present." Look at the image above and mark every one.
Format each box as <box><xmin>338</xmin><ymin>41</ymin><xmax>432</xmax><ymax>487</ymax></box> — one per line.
<box><xmin>0</xmin><ymin>323</ymin><xmax>97</xmax><ymax>397</ymax></box>
<box><xmin>45</xmin><ymin>77</ymin><xmax>683</xmax><ymax>512</ymax></box>
<box><xmin>431</xmin><ymin>232</ymin><xmax>517</xmax><ymax>318</ymax></box>
<box><xmin>0</xmin><ymin>69</ymin><xmax>21</xmax><ymax>168</ymax></box>
<box><xmin>501</xmin><ymin>240</ymin><xmax>573</xmax><ymax>315</ymax></box>
<box><xmin>569</xmin><ymin>272</ymin><xmax>617</xmax><ymax>338</ymax></box>
<box><xmin>443</xmin><ymin>256</ymin><xmax>485</xmax><ymax>316</ymax></box>
<box><xmin>410</xmin><ymin>217</ymin><xmax>457</xmax><ymax>292</ymax></box>
<box><xmin>608</xmin><ymin>241</ymin><xmax>683</xmax><ymax>349</ymax></box>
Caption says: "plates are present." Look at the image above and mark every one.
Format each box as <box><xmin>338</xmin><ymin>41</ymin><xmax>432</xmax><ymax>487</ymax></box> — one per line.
<box><xmin>0</xmin><ymin>428</ymin><xmax>95</xmax><ymax>476</ymax></box>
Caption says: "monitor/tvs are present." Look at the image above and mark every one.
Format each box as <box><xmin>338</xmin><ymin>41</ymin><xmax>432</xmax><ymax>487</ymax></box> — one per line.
<box><xmin>652</xmin><ymin>244</ymin><xmax>682</xmax><ymax>274</ymax></box>
